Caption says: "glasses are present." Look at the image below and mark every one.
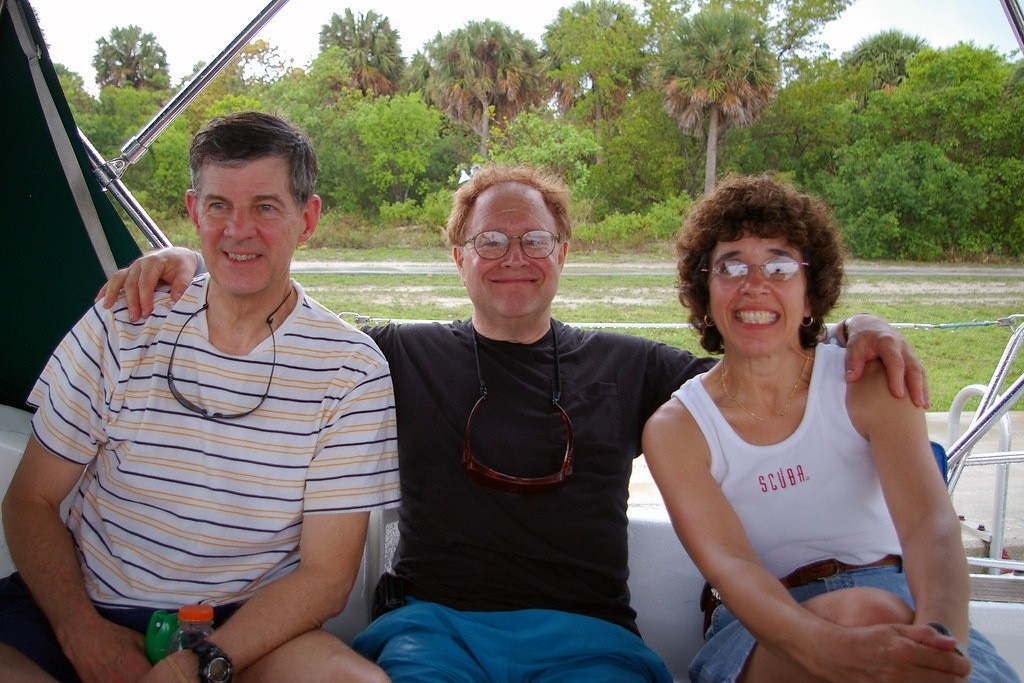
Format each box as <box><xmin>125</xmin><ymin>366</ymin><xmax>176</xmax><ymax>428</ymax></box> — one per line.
<box><xmin>462</xmin><ymin>393</ymin><xmax>576</xmax><ymax>493</ymax></box>
<box><xmin>700</xmin><ymin>255</ymin><xmax>813</xmax><ymax>282</ymax></box>
<box><xmin>460</xmin><ymin>229</ymin><xmax>561</xmax><ymax>261</ymax></box>
<box><xmin>167</xmin><ymin>304</ymin><xmax>276</xmax><ymax>420</ymax></box>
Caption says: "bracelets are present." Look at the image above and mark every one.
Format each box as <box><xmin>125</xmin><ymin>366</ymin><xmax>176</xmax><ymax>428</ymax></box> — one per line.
<box><xmin>926</xmin><ymin>623</ymin><xmax>968</xmax><ymax>658</ymax></box>
<box><xmin>165</xmin><ymin>655</ymin><xmax>188</xmax><ymax>683</ymax></box>
<box><xmin>843</xmin><ymin>313</ymin><xmax>871</xmax><ymax>342</ymax></box>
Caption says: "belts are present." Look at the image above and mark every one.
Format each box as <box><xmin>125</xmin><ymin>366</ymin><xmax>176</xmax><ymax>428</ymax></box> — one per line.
<box><xmin>777</xmin><ymin>555</ymin><xmax>901</xmax><ymax>590</ymax></box>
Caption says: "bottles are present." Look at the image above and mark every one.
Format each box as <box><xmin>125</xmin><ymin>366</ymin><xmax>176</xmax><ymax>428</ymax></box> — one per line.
<box><xmin>164</xmin><ymin>605</ymin><xmax>216</xmax><ymax>658</ymax></box>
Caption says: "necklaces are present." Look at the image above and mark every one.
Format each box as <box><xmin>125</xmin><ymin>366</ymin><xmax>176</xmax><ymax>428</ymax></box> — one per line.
<box><xmin>721</xmin><ymin>349</ymin><xmax>810</xmax><ymax>421</ymax></box>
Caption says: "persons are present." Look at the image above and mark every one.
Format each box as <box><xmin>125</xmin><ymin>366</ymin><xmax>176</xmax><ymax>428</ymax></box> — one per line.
<box><xmin>96</xmin><ymin>163</ymin><xmax>929</xmax><ymax>683</ymax></box>
<box><xmin>642</xmin><ymin>170</ymin><xmax>1023</xmax><ymax>683</ymax></box>
<box><xmin>0</xmin><ymin>111</ymin><xmax>401</xmax><ymax>683</ymax></box>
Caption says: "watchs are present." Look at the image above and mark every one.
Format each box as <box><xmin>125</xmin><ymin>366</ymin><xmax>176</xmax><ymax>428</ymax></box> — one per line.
<box><xmin>183</xmin><ymin>640</ymin><xmax>233</xmax><ymax>683</ymax></box>
